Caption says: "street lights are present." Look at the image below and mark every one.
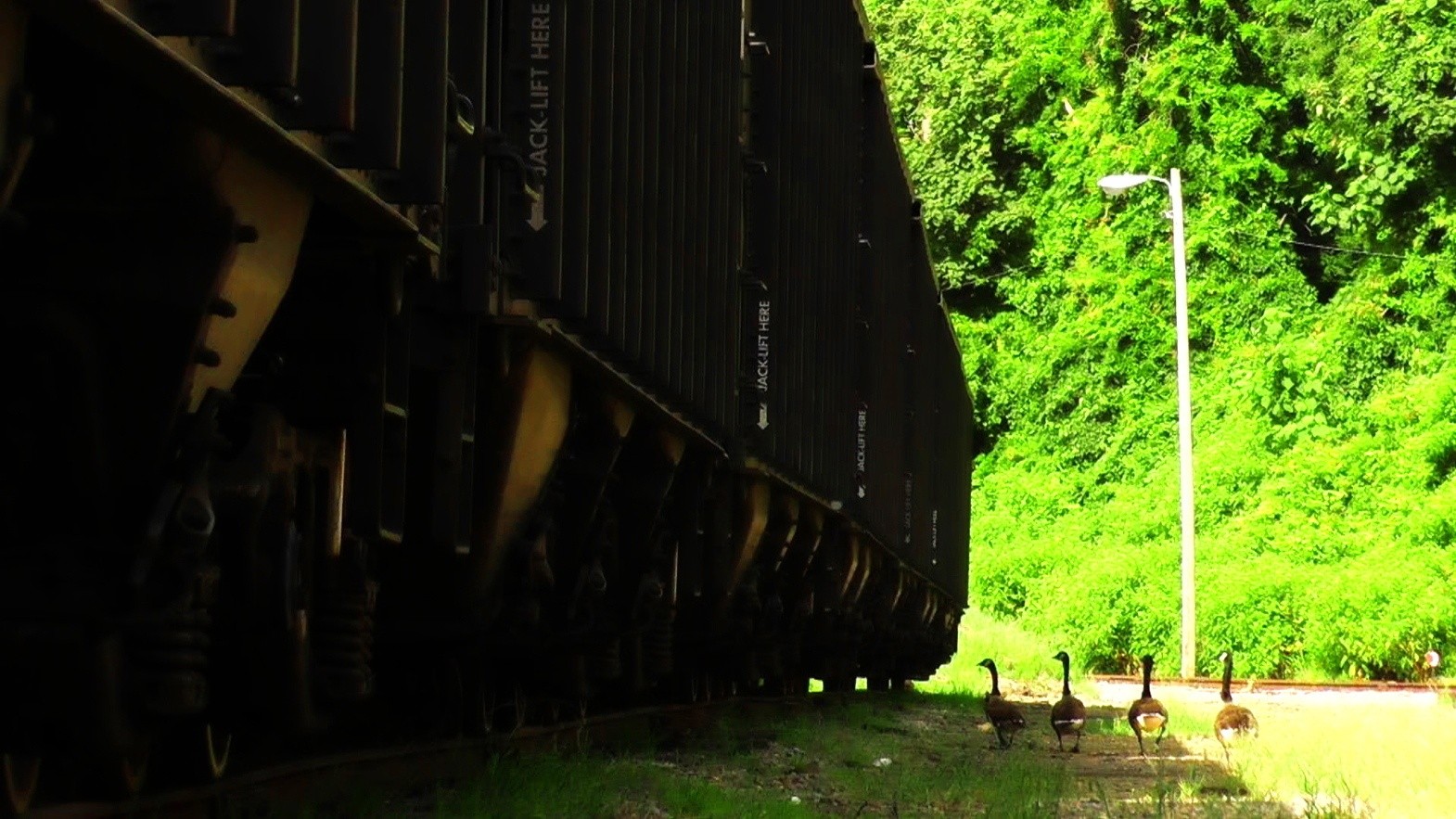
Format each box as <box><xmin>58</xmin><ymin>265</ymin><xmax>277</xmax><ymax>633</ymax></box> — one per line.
<box><xmin>1097</xmin><ymin>160</ymin><xmax>1202</xmax><ymax>679</ymax></box>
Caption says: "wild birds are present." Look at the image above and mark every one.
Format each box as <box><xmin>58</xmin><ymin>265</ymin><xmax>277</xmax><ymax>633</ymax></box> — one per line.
<box><xmin>977</xmin><ymin>657</ymin><xmax>1026</xmax><ymax>750</ymax></box>
<box><xmin>1127</xmin><ymin>653</ymin><xmax>1169</xmax><ymax>755</ymax></box>
<box><xmin>1050</xmin><ymin>651</ymin><xmax>1087</xmax><ymax>755</ymax></box>
<box><xmin>1210</xmin><ymin>650</ymin><xmax>1259</xmax><ymax>760</ymax></box>
